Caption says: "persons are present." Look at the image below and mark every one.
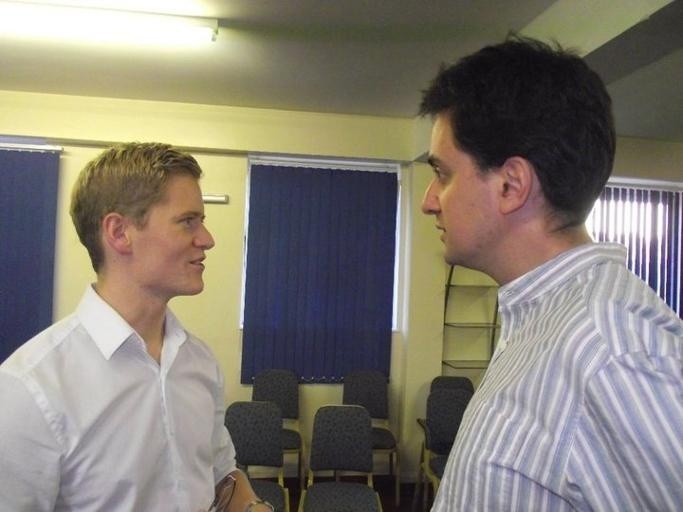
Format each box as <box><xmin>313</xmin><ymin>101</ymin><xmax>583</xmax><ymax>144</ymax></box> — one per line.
<box><xmin>417</xmin><ymin>28</ymin><xmax>683</xmax><ymax>510</ymax></box>
<box><xmin>0</xmin><ymin>142</ymin><xmax>278</xmax><ymax>511</ymax></box>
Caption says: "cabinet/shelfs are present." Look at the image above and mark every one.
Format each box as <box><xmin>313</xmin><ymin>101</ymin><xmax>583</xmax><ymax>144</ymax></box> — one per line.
<box><xmin>444</xmin><ymin>281</ymin><xmax>500</xmax><ymax>370</ymax></box>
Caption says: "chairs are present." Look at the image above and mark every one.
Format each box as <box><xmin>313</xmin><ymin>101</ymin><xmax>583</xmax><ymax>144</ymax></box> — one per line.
<box><xmin>224</xmin><ymin>366</ymin><xmax>473</xmax><ymax>512</ymax></box>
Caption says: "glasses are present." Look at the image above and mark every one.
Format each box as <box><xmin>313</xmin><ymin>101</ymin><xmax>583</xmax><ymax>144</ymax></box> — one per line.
<box><xmin>206</xmin><ymin>473</ymin><xmax>238</xmax><ymax>512</ymax></box>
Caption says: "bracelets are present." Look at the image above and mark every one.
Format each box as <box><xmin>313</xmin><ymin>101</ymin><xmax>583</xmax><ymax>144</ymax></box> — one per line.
<box><xmin>241</xmin><ymin>498</ymin><xmax>275</xmax><ymax>512</ymax></box>
